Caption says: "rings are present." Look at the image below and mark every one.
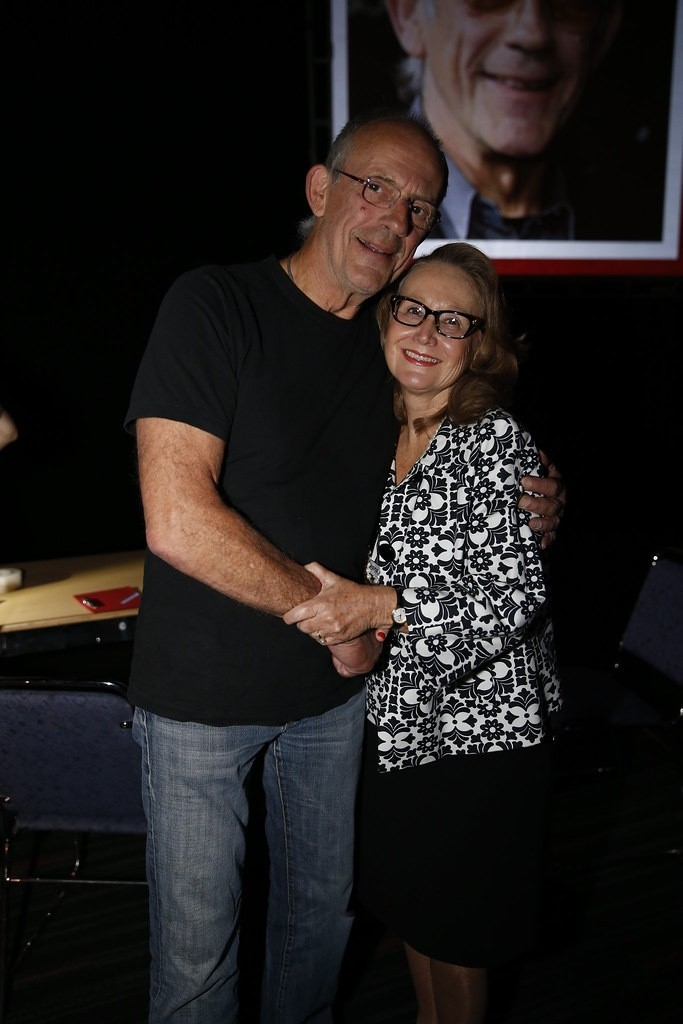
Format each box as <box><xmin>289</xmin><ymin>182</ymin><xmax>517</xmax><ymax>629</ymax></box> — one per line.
<box><xmin>316</xmin><ymin>630</ymin><xmax>326</xmax><ymax>645</ymax></box>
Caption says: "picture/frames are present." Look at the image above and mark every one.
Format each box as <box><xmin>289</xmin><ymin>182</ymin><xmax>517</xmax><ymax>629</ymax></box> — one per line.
<box><xmin>304</xmin><ymin>0</ymin><xmax>683</xmax><ymax>292</ymax></box>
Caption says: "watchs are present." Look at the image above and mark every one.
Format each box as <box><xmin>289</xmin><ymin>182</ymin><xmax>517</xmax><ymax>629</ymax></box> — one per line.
<box><xmin>389</xmin><ymin>586</ymin><xmax>407</xmax><ymax>630</ymax></box>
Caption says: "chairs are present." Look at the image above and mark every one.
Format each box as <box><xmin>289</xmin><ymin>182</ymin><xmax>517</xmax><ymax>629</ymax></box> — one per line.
<box><xmin>558</xmin><ymin>549</ymin><xmax>683</xmax><ymax>858</ymax></box>
<box><xmin>0</xmin><ymin>677</ymin><xmax>148</xmax><ymax>972</ymax></box>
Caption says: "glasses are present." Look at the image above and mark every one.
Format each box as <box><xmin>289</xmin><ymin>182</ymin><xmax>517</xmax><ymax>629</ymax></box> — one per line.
<box><xmin>390</xmin><ymin>295</ymin><xmax>488</xmax><ymax>340</ymax></box>
<box><xmin>332</xmin><ymin>167</ymin><xmax>441</xmax><ymax>231</ymax></box>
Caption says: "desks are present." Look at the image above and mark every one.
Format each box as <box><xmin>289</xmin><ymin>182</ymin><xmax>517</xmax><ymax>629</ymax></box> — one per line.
<box><xmin>0</xmin><ymin>551</ymin><xmax>144</xmax><ymax>634</ymax></box>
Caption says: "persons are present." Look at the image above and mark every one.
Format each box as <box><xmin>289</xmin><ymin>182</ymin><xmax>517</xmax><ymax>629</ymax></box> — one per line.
<box><xmin>385</xmin><ymin>0</ymin><xmax>624</xmax><ymax>242</ymax></box>
<box><xmin>124</xmin><ymin>116</ymin><xmax>568</xmax><ymax>1024</ymax></box>
<box><xmin>283</xmin><ymin>238</ymin><xmax>564</xmax><ymax>1023</ymax></box>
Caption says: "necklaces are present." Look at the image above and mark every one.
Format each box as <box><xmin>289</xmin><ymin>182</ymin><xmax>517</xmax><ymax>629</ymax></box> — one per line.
<box><xmin>287</xmin><ymin>252</ymin><xmax>294</xmax><ymax>282</ymax></box>
